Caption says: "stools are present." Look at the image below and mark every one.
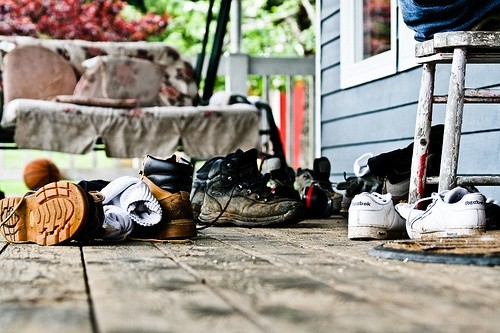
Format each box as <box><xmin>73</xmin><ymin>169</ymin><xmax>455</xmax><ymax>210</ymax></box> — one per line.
<box><xmin>408</xmin><ymin>32</ymin><xmax>500</xmax><ymax>205</ymax></box>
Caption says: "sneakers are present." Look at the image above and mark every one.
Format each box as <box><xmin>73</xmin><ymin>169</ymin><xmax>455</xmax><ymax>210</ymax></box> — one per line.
<box><xmin>128</xmin><ymin>151</ymin><xmax>197</xmax><ymax>239</ymax></box>
<box><xmin>348</xmin><ymin>191</ymin><xmax>407</xmax><ymax>240</ymax></box>
<box><xmin>192</xmin><ymin>148</ymin><xmax>307</xmax><ymax>227</ymax></box>
<box><xmin>0</xmin><ymin>182</ymin><xmax>105</xmax><ymax>246</ymax></box>
<box><xmin>368</xmin><ymin>124</ymin><xmax>444</xmax><ymax>201</ymax></box>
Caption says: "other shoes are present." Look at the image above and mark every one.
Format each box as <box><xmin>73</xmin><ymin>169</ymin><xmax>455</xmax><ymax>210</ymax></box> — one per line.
<box><xmin>406</xmin><ymin>185</ymin><xmax>486</xmax><ymax>239</ymax></box>
<box><xmin>294</xmin><ymin>157</ymin><xmax>384</xmax><ymax>218</ymax></box>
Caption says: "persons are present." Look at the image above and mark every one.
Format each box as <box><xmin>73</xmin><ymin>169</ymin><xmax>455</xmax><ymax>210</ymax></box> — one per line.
<box><xmin>399</xmin><ymin>0</ymin><xmax>500</xmax><ymax>42</ymax></box>
